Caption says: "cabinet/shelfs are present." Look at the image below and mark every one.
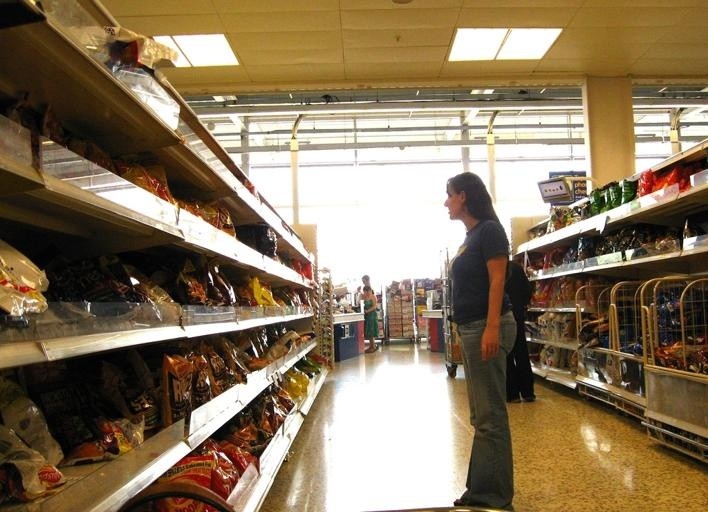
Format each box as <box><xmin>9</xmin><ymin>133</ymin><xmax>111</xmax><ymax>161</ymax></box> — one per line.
<box><xmin>510</xmin><ymin>139</ymin><xmax>707</xmax><ymax>462</ymax></box>
<box><xmin>0</xmin><ymin>1</ymin><xmax>331</xmax><ymax>512</ymax></box>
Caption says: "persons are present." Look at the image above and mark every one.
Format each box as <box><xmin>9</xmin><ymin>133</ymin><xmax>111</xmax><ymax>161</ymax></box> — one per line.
<box><xmin>503</xmin><ymin>261</ymin><xmax>535</xmax><ymax>401</ymax></box>
<box><xmin>444</xmin><ymin>172</ymin><xmax>517</xmax><ymax>512</ymax></box>
<box><xmin>354</xmin><ymin>275</ymin><xmax>379</xmax><ymax>353</ymax></box>
